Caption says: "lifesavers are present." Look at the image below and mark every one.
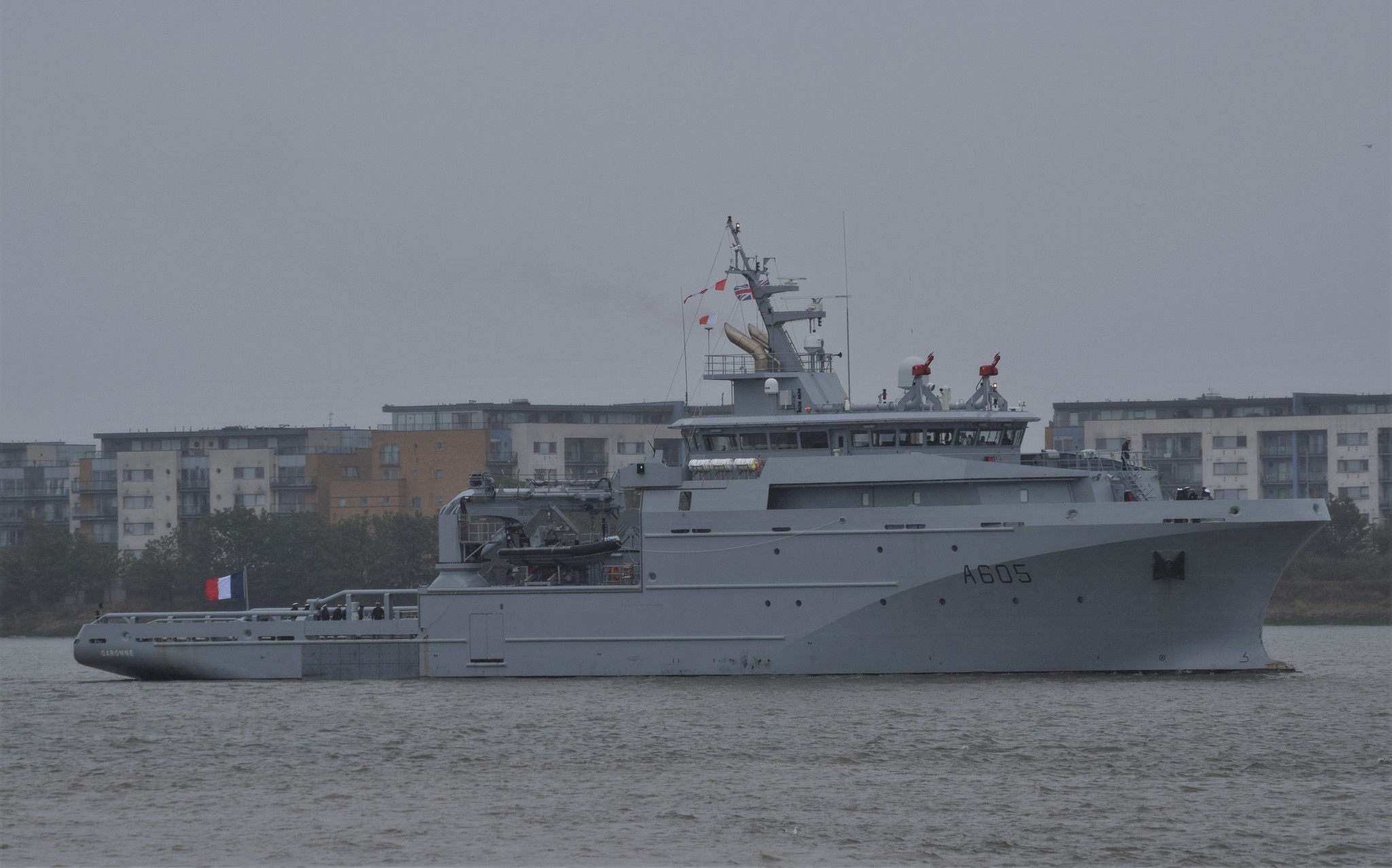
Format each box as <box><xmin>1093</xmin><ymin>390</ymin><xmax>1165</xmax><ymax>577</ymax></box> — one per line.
<box><xmin>611</xmin><ymin>567</ymin><xmax>620</xmax><ymax>580</ymax></box>
<box><xmin>757</xmin><ymin>460</ymin><xmax>764</xmax><ymax>473</ymax></box>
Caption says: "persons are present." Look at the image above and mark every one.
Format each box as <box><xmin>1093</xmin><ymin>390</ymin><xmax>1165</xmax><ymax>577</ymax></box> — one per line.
<box><xmin>290</xmin><ymin>603</ymin><xmax>365</xmax><ymax>637</ymax></box>
<box><xmin>1124</xmin><ymin>490</ymin><xmax>1131</xmax><ymax>502</ymax></box>
<box><xmin>1174</xmin><ymin>486</ymin><xmax>1213</xmax><ymax>524</ymax></box>
<box><xmin>370</xmin><ymin>602</ymin><xmax>385</xmax><ymax>637</ymax></box>
<box><xmin>1121</xmin><ymin>438</ymin><xmax>1132</xmax><ymax>471</ymax></box>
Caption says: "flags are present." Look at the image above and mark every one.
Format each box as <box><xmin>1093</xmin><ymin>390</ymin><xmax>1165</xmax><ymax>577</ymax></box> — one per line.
<box><xmin>205</xmin><ymin>570</ymin><xmax>246</xmax><ymax>601</ymax></box>
<box><xmin>683</xmin><ymin>279</ymin><xmax>727</xmax><ymax>303</ymax></box>
<box><xmin>699</xmin><ymin>312</ymin><xmax>718</xmax><ymax>325</ymax></box>
<box><xmin>734</xmin><ymin>278</ymin><xmax>773</xmax><ymax>301</ymax></box>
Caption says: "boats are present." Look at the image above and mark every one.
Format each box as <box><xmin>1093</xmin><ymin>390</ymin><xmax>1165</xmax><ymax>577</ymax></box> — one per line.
<box><xmin>68</xmin><ymin>212</ymin><xmax>1331</xmax><ymax>682</ymax></box>
